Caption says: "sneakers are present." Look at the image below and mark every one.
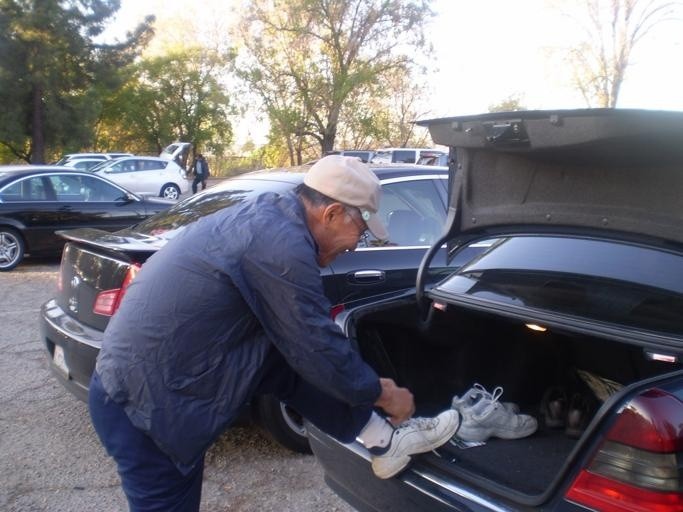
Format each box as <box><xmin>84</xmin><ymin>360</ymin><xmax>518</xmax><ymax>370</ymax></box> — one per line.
<box><xmin>367</xmin><ymin>409</ymin><xmax>462</xmax><ymax>479</ymax></box>
<box><xmin>450</xmin><ymin>383</ymin><xmax>538</xmax><ymax>441</ymax></box>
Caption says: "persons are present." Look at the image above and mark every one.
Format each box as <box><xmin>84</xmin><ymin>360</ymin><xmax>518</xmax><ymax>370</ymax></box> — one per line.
<box><xmin>88</xmin><ymin>154</ymin><xmax>462</xmax><ymax>512</ymax></box>
<box><xmin>187</xmin><ymin>154</ymin><xmax>210</xmax><ymax>194</ymax></box>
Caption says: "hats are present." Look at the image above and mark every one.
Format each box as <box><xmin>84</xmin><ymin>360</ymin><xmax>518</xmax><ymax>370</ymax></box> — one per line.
<box><xmin>303</xmin><ymin>155</ymin><xmax>389</xmax><ymax>241</ymax></box>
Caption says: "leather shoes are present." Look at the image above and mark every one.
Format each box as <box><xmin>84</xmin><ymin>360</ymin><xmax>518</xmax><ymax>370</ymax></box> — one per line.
<box><xmin>540</xmin><ymin>385</ymin><xmax>598</xmax><ymax>437</ymax></box>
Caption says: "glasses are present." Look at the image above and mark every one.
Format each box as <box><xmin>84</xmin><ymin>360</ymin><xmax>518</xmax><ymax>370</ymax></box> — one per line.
<box><xmin>343</xmin><ymin>208</ymin><xmax>370</xmax><ymax>241</ymax></box>
